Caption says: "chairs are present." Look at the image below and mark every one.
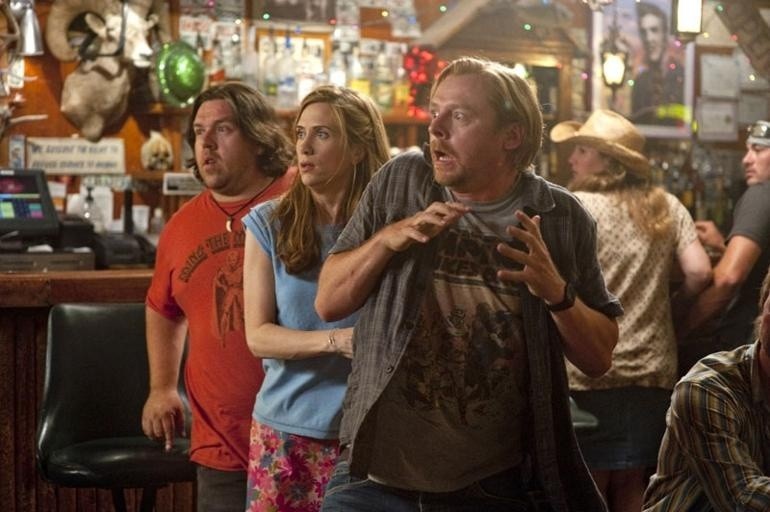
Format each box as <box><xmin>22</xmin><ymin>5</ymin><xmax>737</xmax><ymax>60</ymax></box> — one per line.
<box><xmin>566</xmin><ymin>393</ymin><xmax>600</xmax><ymax>441</ymax></box>
<box><xmin>32</xmin><ymin>303</ymin><xmax>197</xmax><ymax>512</ymax></box>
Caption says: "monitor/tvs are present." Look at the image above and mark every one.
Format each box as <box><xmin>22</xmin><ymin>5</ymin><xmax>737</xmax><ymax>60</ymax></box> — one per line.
<box><xmin>0</xmin><ymin>168</ymin><xmax>60</xmax><ymax>237</ymax></box>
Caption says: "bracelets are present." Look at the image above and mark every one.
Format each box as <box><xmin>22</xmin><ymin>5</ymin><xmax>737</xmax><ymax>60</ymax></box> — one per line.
<box><xmin>326</xmin><ymin>325</ymin><xmax>342</xmax><ymax>352</ymax></box>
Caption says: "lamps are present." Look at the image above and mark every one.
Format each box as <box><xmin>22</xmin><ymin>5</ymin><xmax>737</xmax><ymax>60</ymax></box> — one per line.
<box><xmin>670</xmin><ymin>0</ymin><xmax>702</xmax><ymax>44</ymax></box>
<box><xmin>599</xmin><ymin>50</ymin><xmax>630</xmax><ymax>110</ymax></box>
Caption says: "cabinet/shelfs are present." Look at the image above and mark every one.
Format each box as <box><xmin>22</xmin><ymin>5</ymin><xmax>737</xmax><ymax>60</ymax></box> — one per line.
<box><xmin>411</xmin><ymin>35</ymin><xmax>574</xmax><ymax>189</ymax></box>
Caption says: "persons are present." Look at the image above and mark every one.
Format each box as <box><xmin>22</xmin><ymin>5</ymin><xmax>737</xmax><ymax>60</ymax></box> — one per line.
<box><xmin>627</xmin><ymin>0</ymin><xmax>687</xmax><ymax>124</ymax></box>
<box><xmin>240</xmin><ymin>87</ymin><xmax>400</xmax><ymax>512</ymax></box>
<box><xmin>140</xmin><ymin>84</ymin><xmax>297</xmax><ymax>512</ymax></box>
<box><xmin>679</xmin><ymin>118</ymin><xmax>770</xmax><ymax>338</ymax></box>
<box><xmin>315</xmin><ymin>58</ymin><xmax>620</xmax><ymax>512</ymax></box>
<box><xmin>549</xmin><ymin>109</ymin><xmax>714</xmax><ymax>512</ymax></box>
<box><xmin>644</xmin><ymin>269</ymin><xmax>770</xmax><ymax>512</ymax></box>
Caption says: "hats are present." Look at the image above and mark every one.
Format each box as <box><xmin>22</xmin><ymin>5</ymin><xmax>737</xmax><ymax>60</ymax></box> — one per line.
<box><xmin>157</xmin><ymin>40</ymin><xmax>205</xmax><ymax>109</ymax></box>
<box><xmin>550</xmin><ymin>110</ymin><xmax>649</xmax><ymax>179</ymax></box>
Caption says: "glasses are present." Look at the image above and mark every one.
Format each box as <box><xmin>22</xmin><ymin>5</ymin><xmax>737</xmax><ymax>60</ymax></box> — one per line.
<box><xmin>745</xmin><ymin>124</ymin><xmax>770</xmax><ymax>138</ymax></box>
